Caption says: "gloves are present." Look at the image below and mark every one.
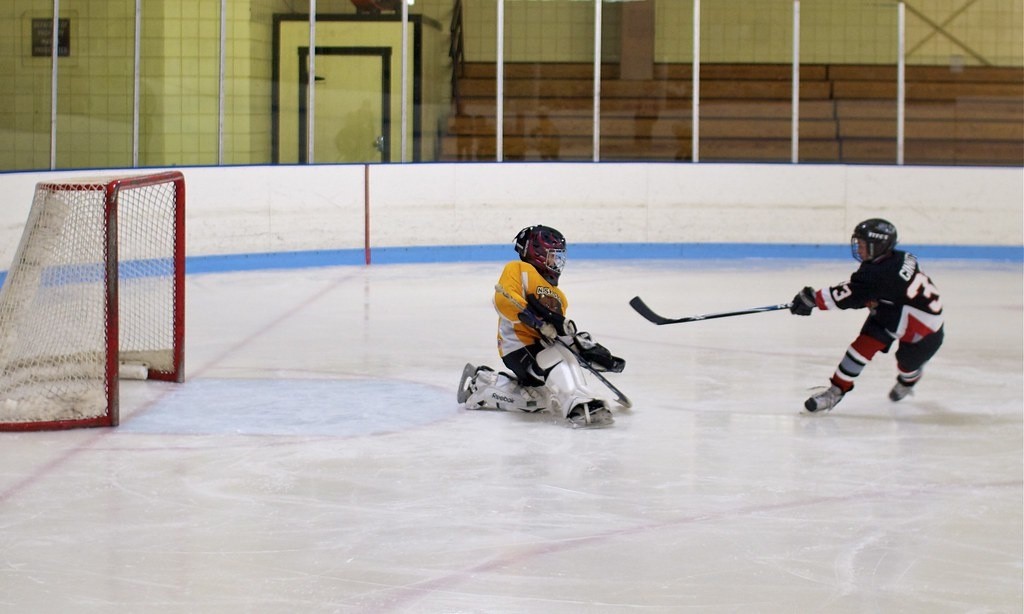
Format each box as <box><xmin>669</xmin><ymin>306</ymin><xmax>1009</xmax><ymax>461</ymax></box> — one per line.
<box><xmin>790</xmin><ymin>287</ymin><xmax>817</xmax><ymax>316</ymax></box>
<box><xmin>536</xmin><ymin>320</ymin><xmax>558</xmax><ymax>344</ymax></box>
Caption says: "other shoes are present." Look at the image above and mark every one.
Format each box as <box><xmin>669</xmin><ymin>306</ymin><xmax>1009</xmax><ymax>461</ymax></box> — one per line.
<box><xmin>457</xmin><ymin>362</ymin><xmax>494</xmax><ymax>406</ymax></box>
<box><xmin>569</xmin><ymin>408</ymin><xmax>615</xmax><ymax>430</ymax></box>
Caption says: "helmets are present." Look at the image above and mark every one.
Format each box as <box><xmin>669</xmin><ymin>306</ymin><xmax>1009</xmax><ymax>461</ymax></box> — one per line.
<box><xmin>850</xmin><ymin>217</ymin><xmax>897</xmax><ymax>262</ymax></box>
<box><xmin>514</xmin><ymin>225</ymin><xmax>567</xmax><ymax>286</ymax></box>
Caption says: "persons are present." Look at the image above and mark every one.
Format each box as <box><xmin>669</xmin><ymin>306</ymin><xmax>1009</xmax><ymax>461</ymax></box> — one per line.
<box><xmin>791</xmin><ymin>218</ymin><xmax>944</xmax><ymax>416</ymax></box>
<box><xmin>456</xmin><ymin>225</ymin><xmax>626</xmax><ymax>428</ymax></box>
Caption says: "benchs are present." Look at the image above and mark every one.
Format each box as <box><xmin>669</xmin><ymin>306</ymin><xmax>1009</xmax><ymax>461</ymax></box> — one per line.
<box><xmin>434</xmin><ymin>61</ymin><xmax>1024</xmax><ymax>166</ymax></box>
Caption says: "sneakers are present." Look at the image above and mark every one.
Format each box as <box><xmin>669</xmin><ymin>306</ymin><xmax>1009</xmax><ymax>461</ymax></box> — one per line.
<box><xmin>888</xmin><ymin>374</ymin><xmax>915</xmax><ymax>403</ymax></box>
<box><xmin>798</xmin><ymin>378</ymin><xmax>854</xmax><ymax>415</ymax></box>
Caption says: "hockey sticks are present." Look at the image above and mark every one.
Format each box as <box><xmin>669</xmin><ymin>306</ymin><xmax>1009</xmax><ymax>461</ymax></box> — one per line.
<box><xmin>492</xmin><ymin>284</ymin><xmax>632</xmax><ymax>410</ymax></box>
<box><xmin>628</xmin><ymin>296</ymin><xmax>794</xmax><ymax>326</ymax></box>
